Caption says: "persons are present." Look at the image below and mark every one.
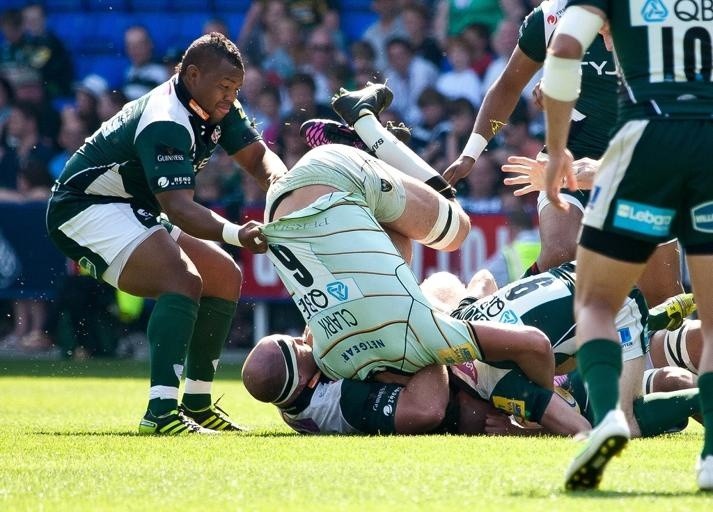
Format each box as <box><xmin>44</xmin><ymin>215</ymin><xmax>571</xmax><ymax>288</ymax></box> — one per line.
<box><xmin>2</xmin><ymin>0</ymin><xmax>188</xmax><ymax>360</ymax></box>
<box><xmin>194</xmin><ymin>0</ymin><xmax>713</xmax><ymax>437</ymax></box>
<box><xmin>46</xmin><ymin>34</ymin><xmax>290</xmax><ymax>433</ymax></box>
<box><xmin>538</xmin><ymin>0</ymin><xmax>712</xmax><ymax>495</ymax></box>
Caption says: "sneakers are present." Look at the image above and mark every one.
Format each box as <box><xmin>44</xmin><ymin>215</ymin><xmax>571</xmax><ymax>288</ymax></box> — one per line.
<box><xmin>299</xmin><ymin>120</ymin><xmax>375</xmax><ymax>156</ymax></box>
<box><xmin>178</xmin><ymin>402</ymin><xmax>246</xmax><ymax>433</ymax></box>
<box><xmin>383</xmin><ymin>122</ymin><xmax>411</xmax><ymax>143</ymax></box>
<box><xmin>698</xmin><ymin>454</ymin><xmax>713</xmax><ymax>490</ymax></box>
<box><xmin>137</xmin><ymin>408</ymin><xmax>217</xmax><ymax>434</ymax></box>
<box><xmin>565</xmin><ymin>410</ymin><xmax>631</xmax><ymax>491</ymax></box>
<box><xmin>332</xmin><ymin>82</ymin><xmax>393</xmax><ymax>125</ymax></box>
<box><xmin>663</xmin><ymin>294</ymin><xmax>696</xmax><ymax>332</ymax></box>
